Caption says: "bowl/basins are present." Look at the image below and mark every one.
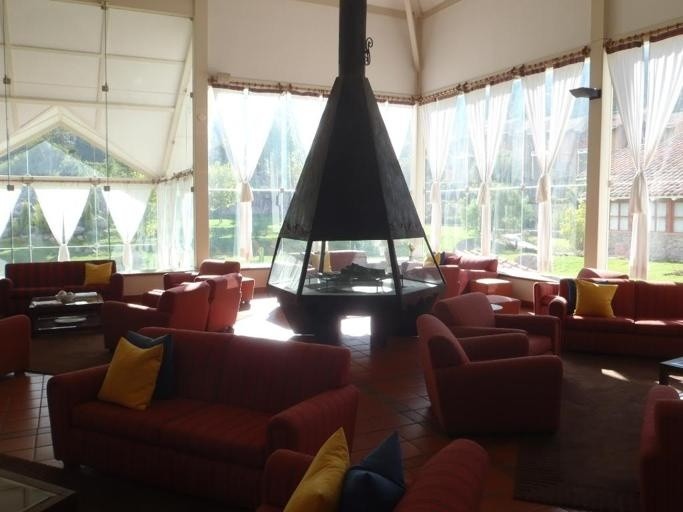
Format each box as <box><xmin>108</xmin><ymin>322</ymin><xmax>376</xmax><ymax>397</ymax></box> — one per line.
<box><xmin>55</xmin><ymin>294</ymin><xmax>77</xmax><ymax>302</ymax></box>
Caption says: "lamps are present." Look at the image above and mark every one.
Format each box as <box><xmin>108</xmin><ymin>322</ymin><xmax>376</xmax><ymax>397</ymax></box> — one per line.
<box><xmin>570</xmin><ymin>87</ymin><xmax>601</xmax><ymax>101</ymax></box>
